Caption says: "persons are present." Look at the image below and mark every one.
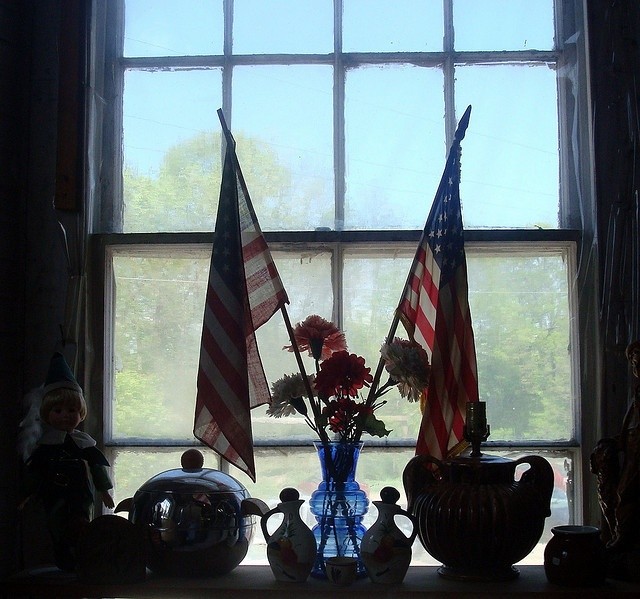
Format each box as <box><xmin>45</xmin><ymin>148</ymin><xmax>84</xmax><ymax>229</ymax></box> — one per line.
<box><xmin>13</xmin><ymin>352</ymin><xmax>114</xmax><ymax>571</ymax></box>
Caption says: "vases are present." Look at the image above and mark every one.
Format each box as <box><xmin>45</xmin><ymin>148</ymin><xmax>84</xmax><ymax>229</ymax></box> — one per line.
<box><xmin>310</xmin><ymin>439</ymin><xmax>367</xmax><ymax>579</ymax></box>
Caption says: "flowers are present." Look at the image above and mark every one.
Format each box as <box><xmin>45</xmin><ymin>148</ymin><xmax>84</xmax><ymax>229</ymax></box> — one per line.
<box><xmin>268</xmin><ymin>316</ymin><xmax>441</xmax><ymax>448</ymax></box>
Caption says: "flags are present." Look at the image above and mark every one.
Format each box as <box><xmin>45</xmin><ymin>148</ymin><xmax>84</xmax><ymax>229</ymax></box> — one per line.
<box><xmin>192</xmin><ymin>144</ymin><xmax>290</xmax><ymax>482</ymax></box>
<box><xmin>390</xmin><ymin>166</ymin><xmax>479</xmax><ymax>477</ymax></box>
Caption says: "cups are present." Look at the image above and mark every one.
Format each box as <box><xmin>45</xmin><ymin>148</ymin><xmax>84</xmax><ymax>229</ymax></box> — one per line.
<box><xmin>325</xmin><ymin>557</ymin><xmax>357</xmax><ymax>587</ymax></box>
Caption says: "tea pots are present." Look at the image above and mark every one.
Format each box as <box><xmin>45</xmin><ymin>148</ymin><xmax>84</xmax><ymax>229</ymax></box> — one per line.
<box><xmin>403</xmin><ymin>451</ymin><xmax>551</xmax><ymax>585</ymax></box>
<box><xmin>360</xmin><ymin>487</ymin><xmax>419</xmax><ymax>584</ymax></box>
<box><xmin>115</xmin><ymin>449</ymin><xmax>267</xmax><ymax>573</ymax></box>
<box><xmin>262</xmin><ymin>488</ymin><xmax>317</xmax><ymax>580</ymax></box>
